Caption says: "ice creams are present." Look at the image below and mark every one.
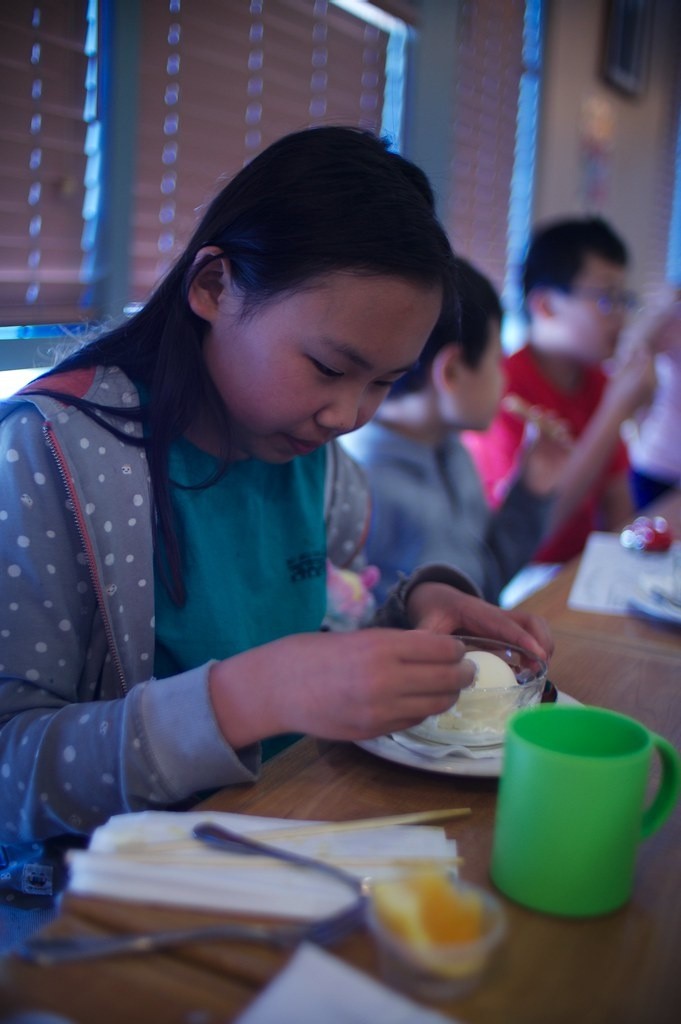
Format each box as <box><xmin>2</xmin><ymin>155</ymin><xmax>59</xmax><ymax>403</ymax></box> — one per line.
<box><xmin>409</xmin><ymin>647</ymin><xmax>527</xmax><ymax>746</ymax></box>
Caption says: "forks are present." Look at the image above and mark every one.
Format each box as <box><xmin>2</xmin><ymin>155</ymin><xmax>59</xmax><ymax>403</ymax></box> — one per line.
<box><xmin>21</xmin><ymin>896</ymin><xmax>372</xmax><ymax>967</ymax></box>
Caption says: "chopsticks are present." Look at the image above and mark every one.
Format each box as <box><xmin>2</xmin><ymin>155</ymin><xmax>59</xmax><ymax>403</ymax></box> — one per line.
<box><xmin>118</xmin><ymin>807</ymin><xmax>473</xmax><ymax>870</ymax></box>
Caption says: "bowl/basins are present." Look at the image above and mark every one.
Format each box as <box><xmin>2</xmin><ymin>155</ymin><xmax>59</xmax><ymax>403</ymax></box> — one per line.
<box><xmin>408</xmin><ymin>634</ymin><xmax>547</xmax><ymax>749</ymax></box>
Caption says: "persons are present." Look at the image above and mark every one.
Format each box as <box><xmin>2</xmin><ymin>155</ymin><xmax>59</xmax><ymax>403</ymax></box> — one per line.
<box><xmin>333</xmin><ymin>249</ymin><xmax>577</xmax><ymax>611</ymax></box>
<box><xmin>457</xmin><ymin>208</ymin><xmax>681</xmax><ymax>609</ymax></box>
<box><xmin>0</xmin><ymin>121</ymin><xmax>557</xmax><ymax>954</ymax></box>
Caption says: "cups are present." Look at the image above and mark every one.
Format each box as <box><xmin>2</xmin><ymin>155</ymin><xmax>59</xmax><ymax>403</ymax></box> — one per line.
<box><xmin>490</xmin><ymin>703</ymin><xmax>681</xmax><ymax>916</ymax></box>
<box><xmin>370</xmin><ymin>884</ymin><xmax>508</xmax><ymax>1001</ymax></box>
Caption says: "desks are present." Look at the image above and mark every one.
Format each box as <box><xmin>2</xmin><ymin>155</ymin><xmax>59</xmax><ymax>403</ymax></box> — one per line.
<box><xmin>0</xmin><ymin>487</ymin><xmax>681</xmax><ymax>1024</ymax></box>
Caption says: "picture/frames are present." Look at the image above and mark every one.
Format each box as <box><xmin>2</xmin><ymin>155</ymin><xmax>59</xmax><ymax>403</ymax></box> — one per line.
<box><xmin>601</xmin><ymin>0</ymin><xmax>653</xmax><ymax>100</ymax></box>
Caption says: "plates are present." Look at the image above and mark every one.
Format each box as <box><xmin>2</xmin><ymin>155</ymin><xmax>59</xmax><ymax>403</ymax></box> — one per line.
<box><xmin>353</xmin><ymin>683</ymin><xmax>583</xmax><ymax>774</ymax></box>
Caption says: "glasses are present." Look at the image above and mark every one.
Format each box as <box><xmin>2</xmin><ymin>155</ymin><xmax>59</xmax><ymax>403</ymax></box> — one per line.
<box><xmin>560</xmin><ymin>288</ymin><xmax>638</xmax><ymax>315</ymax></box>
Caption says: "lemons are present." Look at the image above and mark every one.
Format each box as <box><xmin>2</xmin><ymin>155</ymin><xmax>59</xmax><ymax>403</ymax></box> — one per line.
<box><xmin>368</xmin><ymin>870</ymin><xmax>488</xmax><ymax>980</ymax></box>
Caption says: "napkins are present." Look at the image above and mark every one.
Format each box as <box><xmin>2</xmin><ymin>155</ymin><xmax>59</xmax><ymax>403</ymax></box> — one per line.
<box><xmin>66</xmin><ymin>805</ymin><xmax>457</xmax><ymax>921</ymax></box>
